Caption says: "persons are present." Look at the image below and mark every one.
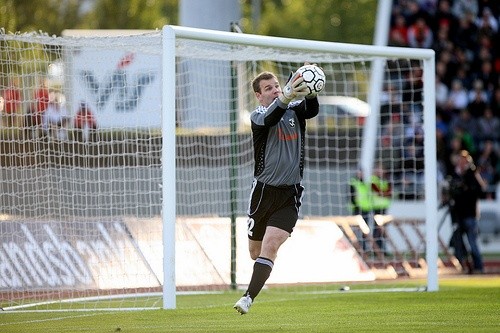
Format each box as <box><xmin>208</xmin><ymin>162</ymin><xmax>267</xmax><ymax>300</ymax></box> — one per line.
<box><xmin>349</xmin><ymin>165</ymin><xmax>391</xmax><ymax>257</ymax></box>
<box><xmin>438</xmin><ymin>150</ymin><xmax>489</xmax><ymax>274</ymax></box>
<box><xmin>2</xmin><ymin>73</ymin><xmax>96</xmax><ymax>141</ymax></box>
<box><xmin>372</xmin><ymin>0</ymin><xmax>500</xmax><ymax>201</ymax></box>
<box><xmin>233</xmin><ymin>62</ymin><xmax>324</xmax><ymax>314</ymax></box>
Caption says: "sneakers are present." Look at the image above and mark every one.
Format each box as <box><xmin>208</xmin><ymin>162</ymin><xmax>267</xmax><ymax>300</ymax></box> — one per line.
<box><xmin>234</xmin><ymin>292</ymin><xmax>253</xmax><ymax>314</ymax></box>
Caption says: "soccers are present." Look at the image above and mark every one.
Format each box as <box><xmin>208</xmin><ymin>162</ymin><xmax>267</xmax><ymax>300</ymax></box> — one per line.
<box><xmin>294</xmin><ymin>65</ymin><xmax>326</xmax><ymax>99</ymax></box>
